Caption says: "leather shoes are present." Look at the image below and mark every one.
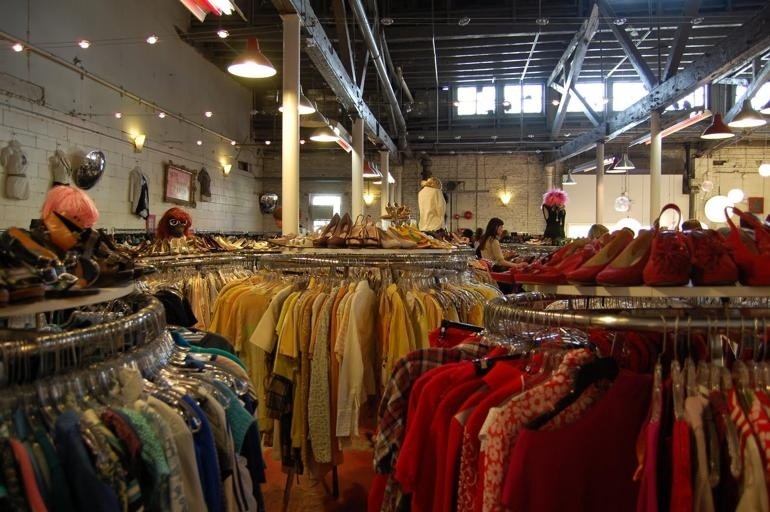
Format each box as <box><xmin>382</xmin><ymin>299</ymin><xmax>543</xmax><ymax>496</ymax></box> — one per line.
<box><xmin>491</xmin><ymin>202</ymin><xmax>770</xmax><ymax>288</ymax></box>
<box><xmin>95</xmin><ymin>228</ymin><xmax>157</xmax><ymax>282</ymax></box>
<box><xmin>0</xmin><ymin>251</ymin><xmax>101</xmax><ymax>301</ymax></box>
<box><xmin>268</xmin><ymin>213</ymin><xmax>447</xmax><ymax>249</ymax></box>
<box><xmin>132</xmin><ymin>233</ymin><xmax>270</xmax><ymax>258</ymax></box>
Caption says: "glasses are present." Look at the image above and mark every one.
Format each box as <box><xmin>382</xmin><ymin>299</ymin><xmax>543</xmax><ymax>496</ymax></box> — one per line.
<box><xmin>170</xmin><ymin>218</ymin><xmax>202</xmax><ymax>227</ymax></box>
<box><xmin>54</xmin><ymin>210</ymin><xmax>92</xmax><ymax>242</ymax></box>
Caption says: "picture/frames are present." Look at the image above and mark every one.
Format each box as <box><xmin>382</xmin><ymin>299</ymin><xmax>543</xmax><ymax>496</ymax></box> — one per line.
<box><xmin>163</xmin><ymin>160</ymin><xmax>198</xmax><ymax>209</ymax></box>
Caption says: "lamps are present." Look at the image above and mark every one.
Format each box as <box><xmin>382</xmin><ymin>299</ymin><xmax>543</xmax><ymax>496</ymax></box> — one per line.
<box><xmin>700</xmin><ymin>55</ymin><xmax>767</xmax><ymax>141</ymax></box>
<box><xmin>226</xmin><ymin>0</ymin><xmax>341</xmax><ymax>144</ymax></box>
<box><xmin>562</xmin><ymin>170</ymin><xmax>577</xmax><ymax>186</ymax></box>
<box><xmin>604</xmin><ymin>151</ymin><xmax>636</xmax><ymax>173</ymax></box>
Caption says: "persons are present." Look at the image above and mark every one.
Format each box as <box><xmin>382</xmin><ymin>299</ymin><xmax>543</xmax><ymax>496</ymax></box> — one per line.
<box><xmin>47</xmin><ymin>150</ymin><xmax>72</xmax><ymax>188</ymax></box>
<box><xmin>197</xmin><ymin>166</ymin><xmax>213</xmax><ymax>202</ymax></box>
<box><xmin>0</xmin><ymin>138</ymin><xmax>32</xmax><ymax>200</ymax></box>
<box><xmin>128</xmin><ymin>165</ymin><xmax>150</xmax><ymax>221</ymax></box>
<box><xmin>542</xmin><ymin>191</ymin><xmax>568</xmax><ymax>238</ymax></box>
<box><xmin>418</xmin><ymin>176</ymin><xmax>445</xmax><ymax>246</ymax></box>
<box><xmin>475</xmin><ymin>217</ymin><xmax>531</xmax><ymax>295</ymax></box>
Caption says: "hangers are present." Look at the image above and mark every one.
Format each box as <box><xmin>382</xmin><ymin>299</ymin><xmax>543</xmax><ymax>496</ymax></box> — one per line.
<box><xmin>0</xmin><ymin>251</ymin><xmax>770</xmax><ymax>490</ymax></box>
